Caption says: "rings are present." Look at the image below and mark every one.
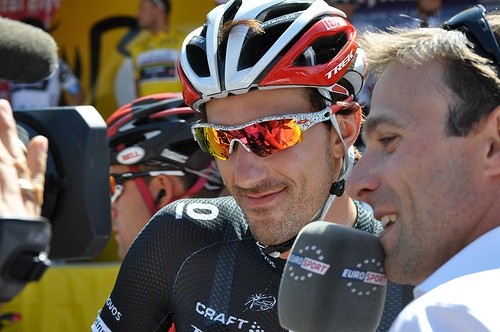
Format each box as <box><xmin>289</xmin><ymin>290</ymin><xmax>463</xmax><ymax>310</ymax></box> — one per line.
<box><xmin>19</xmin><ymin>176</ymin><xmax>43</xmax><ymax>208</ymax></box>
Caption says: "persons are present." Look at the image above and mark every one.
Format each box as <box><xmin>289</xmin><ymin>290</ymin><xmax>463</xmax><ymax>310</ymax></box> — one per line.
<box><xmin>325</xmin><ymin>0</ymin><xmax>444</xmax><ymax>155</ymax></box>
<box><xmin>125</xmin><ymin>0</ymin><xmax>190</xmax><ymax>98</ymax></box>
<box><xmin>90</xmin><ymin>0</ymin><xmax>415</xmax><ymax>332</ymax></box>
<box><xmin>8</xmin><ymin>18</ymin><xmax>85</xmax><ymax>109</ymax></box>
<box><xmin>0</xmin><ymin>98</ymin><xmax>52</xmax><ymax>302</ymax></box>
<box><xmin>106</xmin><ymin>92</ymin><xmax>231</xmax><ymax>262</ymax></box>
<box><xmin>345</xmin><ymin>4</ymin><xmax>500</xmax><ymax>332</ymax></box>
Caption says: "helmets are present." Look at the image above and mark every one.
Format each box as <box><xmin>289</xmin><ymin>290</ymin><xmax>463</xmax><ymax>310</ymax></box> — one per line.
<box><xmin>177</xmin><ymin>0</ymin><xmax>369</xmax><ymax>113</ymax></box>
<box><xmin>106</xmin><ymin>92</ymin><xmax>225</xmax><ymax>196</ymax></box>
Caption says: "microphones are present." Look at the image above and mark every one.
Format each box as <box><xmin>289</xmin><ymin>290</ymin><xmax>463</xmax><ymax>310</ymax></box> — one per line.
<box><xmin>277</xmin><ymin>221</ymin><xmax>386</xmax><ymax>332</ymax></box>
<box><xmin>0</xmin><ymin>17</ymin><xmax>59</xmax><ymax>84</ymax></box>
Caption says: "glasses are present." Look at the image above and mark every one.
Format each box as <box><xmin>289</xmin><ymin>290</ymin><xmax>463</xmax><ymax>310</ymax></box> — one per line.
<box><xmin>443</xmin><ymin>4</ymin><xmax>500</xmax><ymax>66</ymax></box>
<box><xmin>108</xmin><ymin>171</ymin><xmax>185</xmax><ymax>203</ymax></box>
<box><xmin>192</xmin><ymin>94</ymin><xmax>354</xmax><ymax>161</ymax></box>
